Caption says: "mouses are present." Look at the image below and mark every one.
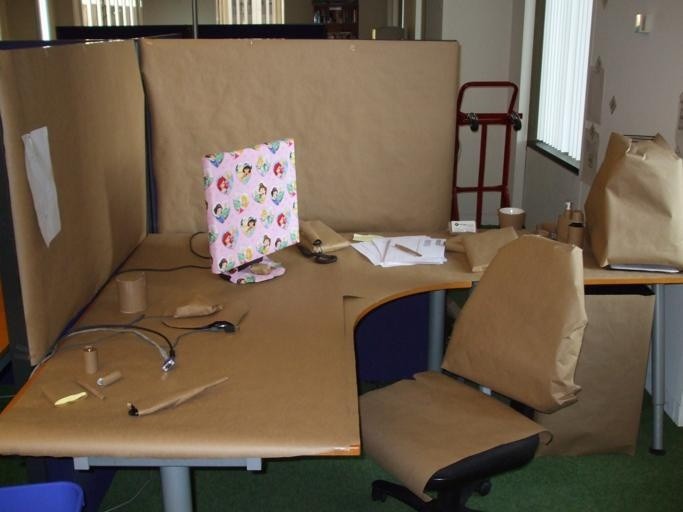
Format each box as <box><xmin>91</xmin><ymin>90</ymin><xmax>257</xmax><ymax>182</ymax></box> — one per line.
<box><xmin>206</xmin><ymin>320</ymin><xmax>236</xmax><ymax>334</ymax></box>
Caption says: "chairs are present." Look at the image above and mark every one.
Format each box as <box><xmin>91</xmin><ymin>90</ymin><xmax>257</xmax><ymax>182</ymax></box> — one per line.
<box><xmin>359</xmin><ymin>237</ymin><xmax>582</xmax><ymax>511</ymax></box>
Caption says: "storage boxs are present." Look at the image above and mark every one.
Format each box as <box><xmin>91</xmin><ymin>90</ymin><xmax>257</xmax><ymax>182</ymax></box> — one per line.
<box><xmin>509</xmin><ymin>283</ymin><xmax>654</xmax><ymax>458</ymax></box>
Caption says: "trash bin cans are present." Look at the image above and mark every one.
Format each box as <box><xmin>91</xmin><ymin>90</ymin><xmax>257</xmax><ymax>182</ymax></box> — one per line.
<box><xmin>0</xmin><ymin>481</ymin><xmax>85</xmax><ymax>512</ymax></box>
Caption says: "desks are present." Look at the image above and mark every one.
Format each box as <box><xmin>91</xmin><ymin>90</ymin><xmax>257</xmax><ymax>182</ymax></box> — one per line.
<box><xmin>0</xmin><ymin>233</ymin><xmax>681</xmax><ymax>512</ymax></box>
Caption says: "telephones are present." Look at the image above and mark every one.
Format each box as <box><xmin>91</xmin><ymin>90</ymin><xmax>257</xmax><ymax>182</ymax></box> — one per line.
<box><xmin>297</xmin><ymin>219</ymin><xmax>350</xmax><ymax>254</ymax></box>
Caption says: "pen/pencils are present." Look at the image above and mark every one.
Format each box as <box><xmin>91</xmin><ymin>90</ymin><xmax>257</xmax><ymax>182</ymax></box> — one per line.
<box><xmin>77</xmin><ymin>380</ymin><xmax>104</xmax><ymax>400</ymax></box>
<box><xmin>382</xmin><ymin>240</ymin><xmax>390</xmax><ymax>261</ymax></box>
<box><xmin>395</xmin><ymin>244</ymin><xmax>422</xmax><ymax>257</ymax></box>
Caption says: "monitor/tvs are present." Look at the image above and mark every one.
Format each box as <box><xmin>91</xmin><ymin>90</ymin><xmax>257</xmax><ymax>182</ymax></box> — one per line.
<box><xmin>202</xmin><ymin>137</ymin><xmax>301</xmax><ymax>286</ymax></box>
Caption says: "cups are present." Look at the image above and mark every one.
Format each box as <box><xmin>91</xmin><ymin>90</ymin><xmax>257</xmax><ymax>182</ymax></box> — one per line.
<box><xmin>568</xmin><ymin>223</ymin><xmax>585</xmax><ymax>250</ymax></box>
<box><xmin>497</xmin><ymin>207</ymin><xmax>525</xmax><ymax>228</ymax></box>
<box><xmin>115</xmin><ymin>270</ymin><xmax>147</xmax><ymax>316</ymax></box>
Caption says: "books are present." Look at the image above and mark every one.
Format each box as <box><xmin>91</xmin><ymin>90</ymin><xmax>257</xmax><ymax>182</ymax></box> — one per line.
<box><xmin>379</xmin><ymin>236</ymin><xmax>447</xmax><ymax>268</ymax></box>
<box><xmin>461</xmin><ymin>225</ymin><xmax>520</xmax><ymax>273</ymax></box>
<box><xmin>443</xmin><ymin>230</ymin><xmax>479</xmax><ymax>254</ymax></box>
